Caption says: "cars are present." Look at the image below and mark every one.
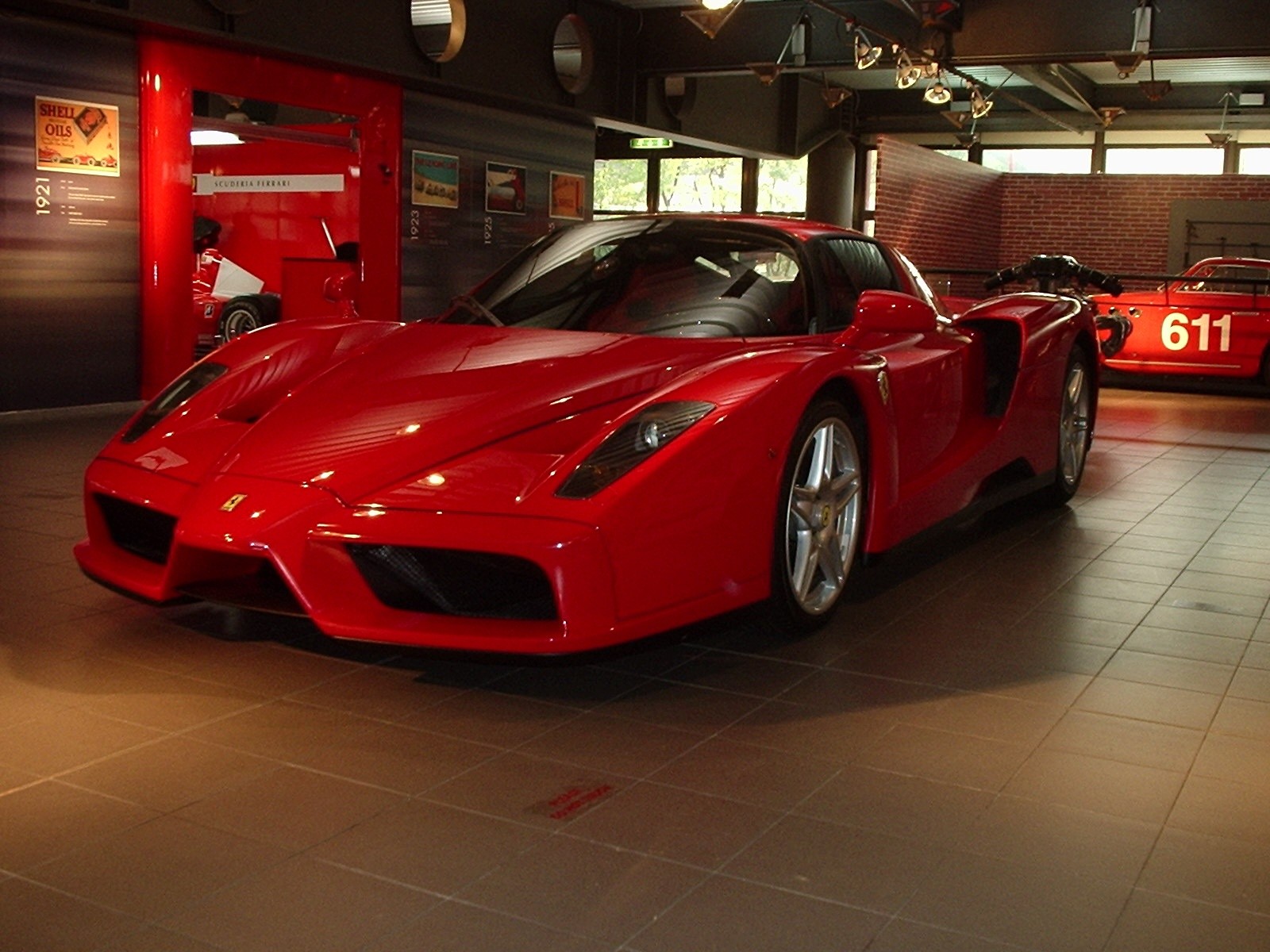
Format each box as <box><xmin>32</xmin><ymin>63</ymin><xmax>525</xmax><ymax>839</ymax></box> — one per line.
<box><xmin>1087</xmin><ymin>255</ymin><xmax>1270</xmax><ymax>399</ymax></box>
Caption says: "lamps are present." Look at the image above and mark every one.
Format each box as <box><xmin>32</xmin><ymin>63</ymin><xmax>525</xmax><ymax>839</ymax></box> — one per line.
<box><xmin>893</xmin><ymin>43</ymin><xmax>922</xmax><ymax>89</ymax></box>
<box><xmin>966</xmin><ymin>81</ymin><xmax>994</xmax><ymax>120</ymax></box>
<box><xmin>850</xmin><ymin>22</ymin><xmax>882</xmax><ymax>69</ymax></box>
<box><xmin>1138</xmin><ymin>59</ymin><xmax>1174</xmax><ymax>102</ymax></box>
<box><xmin>924</xmin><ymin>60</ymin><xmax>952</xmax><ymax>104</ymax></box>
<box><xmin>955</xmin><ymin>116</ymin><xmax>980</xmax><ymax>148</ymax></box>
<box><xmin>940</xmin><ymin>72</ymin><xmax>1013</xmax><ymax>127</ymax></box>
<box><xmin>1205</xmin><ymin>91</ymin><xmax>1238</xmax><ymax>148</ymax></box>
<box><xmin>1108</xmin><ymin>1</ymin><xmax>1149</xmax><ymax>75</ymax></box>
<box><xmin>744</xmin><ymin>3</ymin><xmax>809</xmax><ymax>86</ymax></box>
<box><xmin>820</xmin><ymin>71</ymin><xmax>852</xmax><ymax>106</ymax></box>
<box><xmin>1048</xmin><ymin>64</ymin><xmax>1127</xmax><ymax>128</ymax></box>
<box><xmin>681</xmin><ymin>0</ymin><xmax>744</xmax><ymax>40</ymax></box>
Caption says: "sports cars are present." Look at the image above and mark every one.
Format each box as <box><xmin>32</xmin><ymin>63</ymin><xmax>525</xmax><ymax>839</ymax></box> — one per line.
<box><xmin>71</xmin><ymin>214</ymin><xmax>1132</xmax><ymax>656</ymax></box>
